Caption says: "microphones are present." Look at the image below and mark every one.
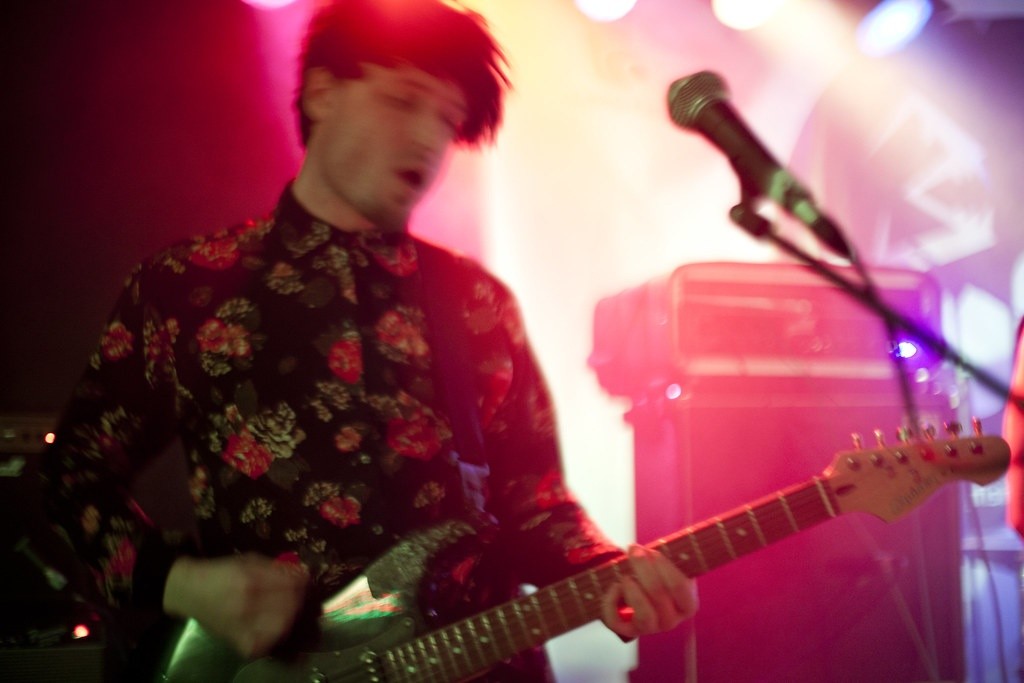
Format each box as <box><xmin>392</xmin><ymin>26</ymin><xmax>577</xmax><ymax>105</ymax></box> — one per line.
<box><xmin>666</xmin><ymin>71</ymin><xmax>854</xmax><ymax>257</ymax></box>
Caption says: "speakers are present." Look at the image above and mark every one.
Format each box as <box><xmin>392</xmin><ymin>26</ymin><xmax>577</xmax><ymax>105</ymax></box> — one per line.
<box><xmin>623</xmin><ymin>395</ymin><xmax>970</xmax><ymax>683</ymax></box>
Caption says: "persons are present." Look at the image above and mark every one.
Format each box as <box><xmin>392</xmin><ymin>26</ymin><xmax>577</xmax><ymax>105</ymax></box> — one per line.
<box><xmin>0</xmin><ymin>0</ymin><xmax>698</xmax><ymax>683</ymax></box>
<box><xmin>1001</xmin><ymin>321</ymin><xmax>1024</xmax><ymax>537</ymax></box>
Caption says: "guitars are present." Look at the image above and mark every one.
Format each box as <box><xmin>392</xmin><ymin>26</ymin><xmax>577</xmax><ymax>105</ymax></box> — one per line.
<box><xmin>167</xmin><ymin>420</ymin><xmax>1011</xmax><ymax>682</ymax></box>
